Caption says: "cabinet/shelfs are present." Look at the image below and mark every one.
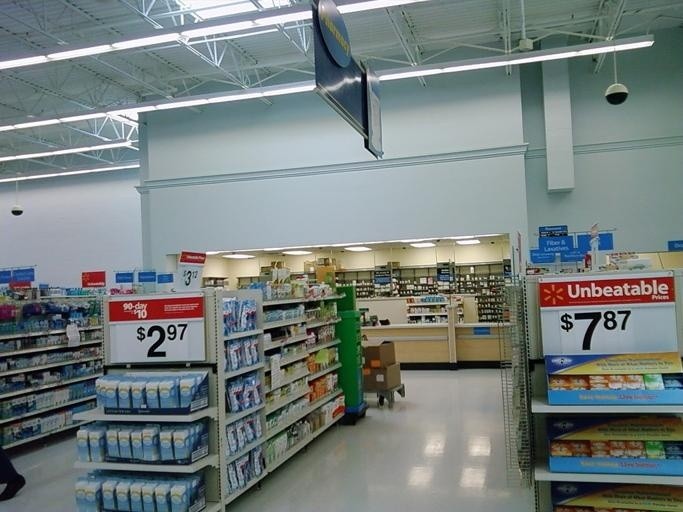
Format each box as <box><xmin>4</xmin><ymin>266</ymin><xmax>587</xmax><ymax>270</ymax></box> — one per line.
<box><xmin>512</xmin><ymin>258</ymin><xmax>683</xmax><ymax>512</ymax></box>
<box><xmin>1</xmin><ymin>283</ymin><xmax>102</xmax><ymax>449</ymax></box>
<box><xmin>98</xmin><ymin>299</ymin><xmax>219</xmax><ymax>512</ymax></box>
<box><xmin>215</xmin><ymin>289</ymin><xmax>347</xmax><ymax>496</ymax></box>
<box><xmin>351</xmin><ymin>263</ymin><xmax>507</xmax><ymax>328</ymax></box>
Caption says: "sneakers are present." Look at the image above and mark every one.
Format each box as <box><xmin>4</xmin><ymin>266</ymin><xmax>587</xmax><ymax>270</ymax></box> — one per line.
<box><xmin>0</xmin><ymin>472</ymin><xmax>26</xmax><ymax>501</ymax></box>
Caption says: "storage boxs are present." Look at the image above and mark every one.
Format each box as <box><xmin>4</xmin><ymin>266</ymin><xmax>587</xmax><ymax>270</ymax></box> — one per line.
<box><xmin>365</xmin><ymin>338</ymin><xmax>396</xmax><ymax>367</ymax></box>
<box><xmin>362</xmin><ymin>362</ymin><xmax>401</xmax><ymax>392</ymax></box>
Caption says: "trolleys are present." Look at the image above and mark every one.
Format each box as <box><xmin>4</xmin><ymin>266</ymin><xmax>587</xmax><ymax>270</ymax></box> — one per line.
<box><xmin>363</xmin><ymin>383</ymin><xmax>406</xmax><ymax>409</ymax></box>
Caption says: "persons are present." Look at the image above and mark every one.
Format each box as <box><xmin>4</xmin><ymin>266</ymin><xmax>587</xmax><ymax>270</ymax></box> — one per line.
<box><xmin>0</xmin><ymin>446</ymin><xmax>25</xmax><ymax>502</ymax></box>
